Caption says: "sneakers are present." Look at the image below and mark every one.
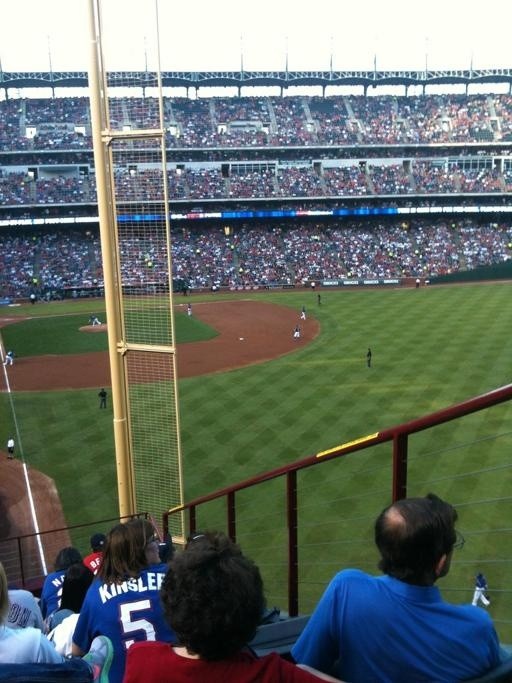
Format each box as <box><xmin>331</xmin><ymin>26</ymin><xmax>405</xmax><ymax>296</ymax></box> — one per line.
<box><xmin>80</xmin><ymin>635</ymin><xmax>115</xmax><ymax>683</ymax></box>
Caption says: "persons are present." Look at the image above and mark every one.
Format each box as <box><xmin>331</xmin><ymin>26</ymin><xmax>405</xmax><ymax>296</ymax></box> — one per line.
<box><xmin>293</xmin><ymin>324</ymin><xmax>301</xmax><ymax>341</ymax></box>
<box><xmin>472</xmin><ymin>572</ymin><xmax>490</xmax><ymax>607</ymax></box>
<box><xmin>0</xmin><ymin>519</ymin><xmax>325</xmax><ymax>683</ymax></box>
<box><xmin>98</xmin><ymin>388</ymin><xmax>107</xmax><ymax>408</ymax></box>
<box><xmin>367</xmin><ymin>348</ymin><xmax>371</xmax><ymax>367</ymax></box>
<box><xmin>301</xmin><ymin>306</ymin><xmax>306</xmax><ymax>320</ymax></box>
<box><xmin>3</xmin><ymin>349</ymin><xmax>17</xmax><ymax>365</ymax></box>
<box><xmin>186</xmin><ymin>303</ymin><xmax>192</xmax><ymax>320</ymax></box>
<box><xmin>290</xmin><ymin>494</ymin><xmax>499</xmax><ymax>682</ymax></box>
<box><xmin>1</xmin><ymin>94</ymin><xmax>512</xmax><ymax>305</ymax></box>
<box><xmin>88</xmin><ymin>312</ymin><xmax>101</xmax><ymax>326</ymax></box>
<box><xmin>7</xmin><ymin>437</ymin><xmax>14</xmax><ymax>459</ymax></box>
